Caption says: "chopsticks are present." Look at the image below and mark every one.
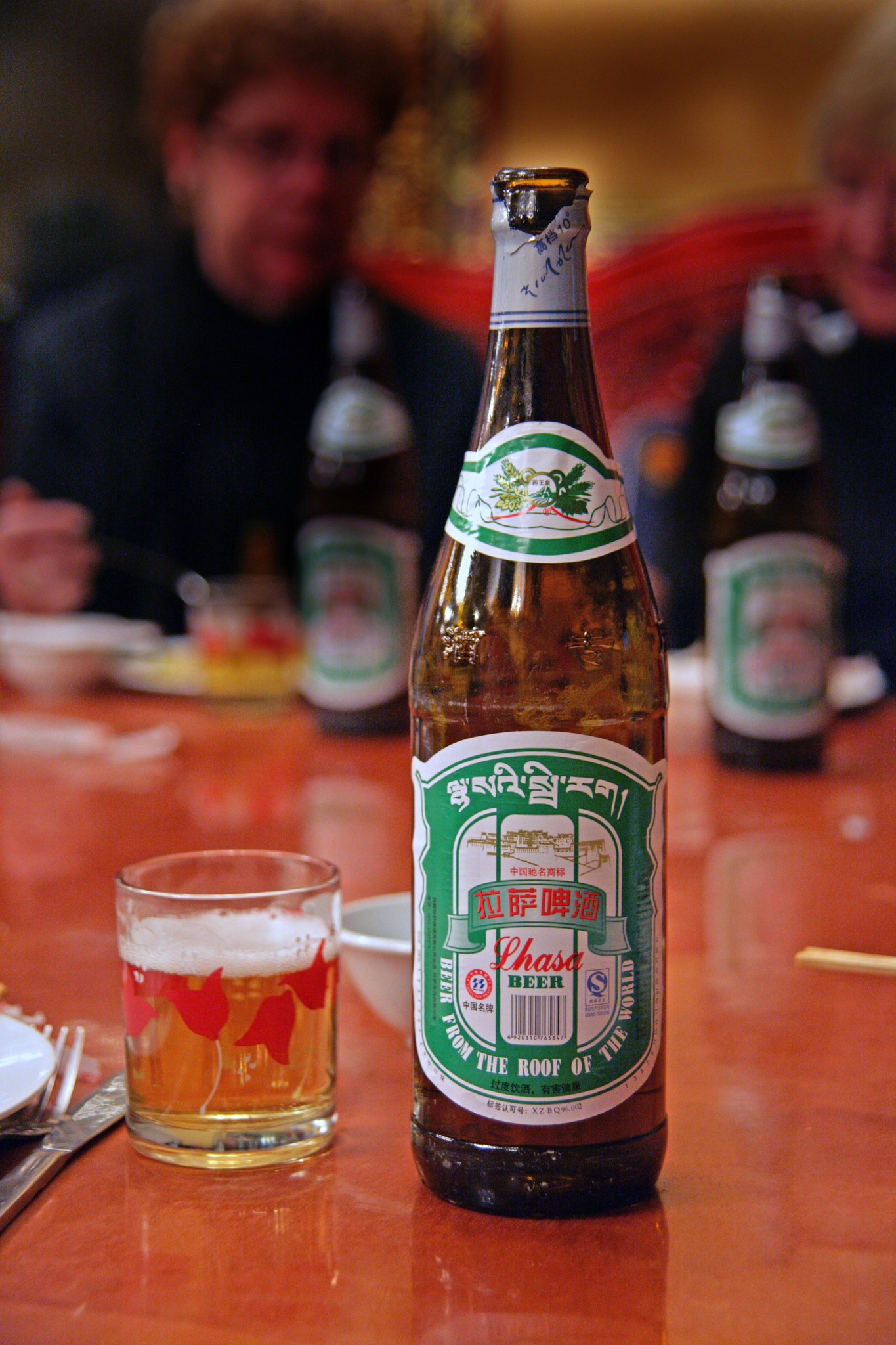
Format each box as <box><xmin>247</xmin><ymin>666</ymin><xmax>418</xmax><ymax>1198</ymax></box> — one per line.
<box><xmin>793</xmin><ymin>946</ymin><xmax>896</xmax><ymax>978</ymax></box>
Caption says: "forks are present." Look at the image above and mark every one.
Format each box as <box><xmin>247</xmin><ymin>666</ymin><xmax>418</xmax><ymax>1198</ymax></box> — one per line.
<box><xmin>1</xmin><ymin>1024</ymin><xmax>86</xmax><ymax>1136</ymax></box>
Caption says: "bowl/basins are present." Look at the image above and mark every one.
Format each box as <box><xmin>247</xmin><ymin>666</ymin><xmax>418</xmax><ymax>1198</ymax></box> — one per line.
<box><xmin>339</xmin><ymin>892</ymin><xmax>414</xmax><ymax>1031</ymax></box>
<box><xmin>1</xmin><ymin>609</ymin><xmax>156</xmax><ymax>697</ymax></box>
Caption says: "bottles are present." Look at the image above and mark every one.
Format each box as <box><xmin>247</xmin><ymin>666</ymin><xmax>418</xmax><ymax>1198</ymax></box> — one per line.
<box><xmin>405</xmin><ymin>161</ymin><xmax>668</xmax><ymax>1219</ymax></box>
<box><xmin>703</xmin><ymin>276</ymin><xmax>841</xmax><ymax>774</ymax></box>
<box><xmin>300</xmin><ymin>286</ymin><xmax>423</xmax><ymax>737</ymax></box>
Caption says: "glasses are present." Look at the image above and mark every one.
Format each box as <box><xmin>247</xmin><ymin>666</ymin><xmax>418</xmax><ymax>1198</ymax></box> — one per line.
<box><xmin>208</xmin><ymin>116</ymin><xmax>381</xmax><ymax>178</ymax></box>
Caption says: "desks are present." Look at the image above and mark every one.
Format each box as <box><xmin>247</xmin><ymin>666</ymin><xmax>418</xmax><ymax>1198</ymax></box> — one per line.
<box><xmin>0</xmin><ymin>643</ymin><xmax>896</xmax><ymax>1345</ymax></box>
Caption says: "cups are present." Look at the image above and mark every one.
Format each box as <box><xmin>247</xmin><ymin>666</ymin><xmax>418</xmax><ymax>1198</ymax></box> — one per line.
<box><xmin>115</xmin><ymin>849</ymin><xmax>342</xmax><ymax>1172</ymax></box>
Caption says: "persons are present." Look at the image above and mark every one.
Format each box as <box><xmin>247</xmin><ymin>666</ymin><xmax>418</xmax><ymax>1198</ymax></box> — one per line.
<box><xmin>0</xmin><ymin>0</ymin><xmax>491</xmax><ymax>639</ymax></box>
<box><xmin>665</xmin><ymin>14</ymin><xmax>895</xmax><ymax>695</ymax></box>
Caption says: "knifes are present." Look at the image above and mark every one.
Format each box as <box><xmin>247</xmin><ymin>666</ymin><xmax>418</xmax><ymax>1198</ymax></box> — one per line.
<box><xmin>0</xmin><ymin>1065</ymin><xmax>127</xmax><ymax>1233</ymax></box>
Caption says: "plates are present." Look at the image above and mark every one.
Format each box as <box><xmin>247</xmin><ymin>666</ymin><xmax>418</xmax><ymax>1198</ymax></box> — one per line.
<box><xmin>111</xmin><ymin>635</ymin><xmax>295</xmax><ymax>698</ymax></box>
<box><xmin>0</xmin><ymin>1013</ymin><xmax>56</xmax><ymax>1122</ymax></box>
